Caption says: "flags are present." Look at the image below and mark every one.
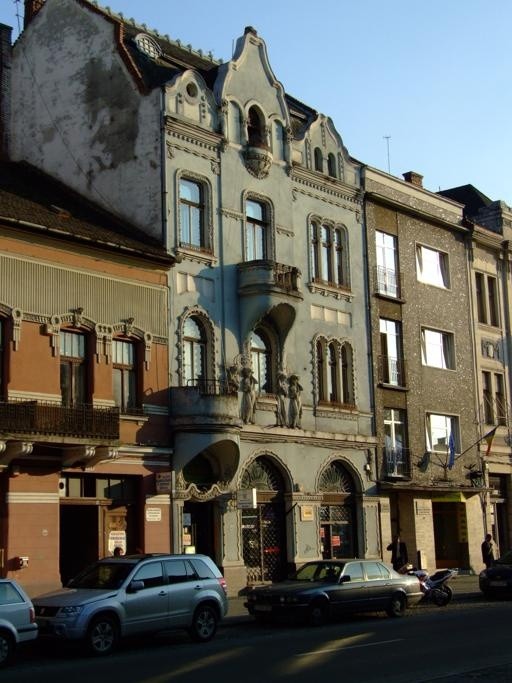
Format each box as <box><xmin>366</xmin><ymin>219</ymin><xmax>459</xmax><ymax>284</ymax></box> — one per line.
<box><xmin>484</xmin><ymin>428</ymin><xmax>495</xmax><ymax>455</ymax></box>
<box><xmin>448</xmin><ymin>433</ymin><xmax>455</xmax><ymax>470</ymax></box>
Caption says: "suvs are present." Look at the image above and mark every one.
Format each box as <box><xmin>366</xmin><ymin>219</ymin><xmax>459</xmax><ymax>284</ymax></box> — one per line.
<box><xmin>32</xmin><ymin>552</ymin><xmax>230</xmax><ymax>655</ymax></box>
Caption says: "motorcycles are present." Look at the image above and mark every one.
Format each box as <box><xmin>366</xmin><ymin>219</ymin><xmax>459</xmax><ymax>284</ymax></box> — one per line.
<box><xmin>398</xmin><ymin>562</ymin><xmax>459</xmax><ymax>607</ymax></box>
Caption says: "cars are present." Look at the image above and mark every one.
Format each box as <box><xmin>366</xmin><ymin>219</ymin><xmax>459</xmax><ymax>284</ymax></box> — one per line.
<box><xmin>478</xmin><ymin>551</ymin><xmax>512</xmax><ymax>601</ymax></box>
<box><xmin>245</xmin><ymin>557</ymin><xmax>426</xmax><ymax>626</ymax></box>
<box><xmin>0</xmin><ymin>578</ymin><xmax>38</xmax><ymax>666</ymax></box>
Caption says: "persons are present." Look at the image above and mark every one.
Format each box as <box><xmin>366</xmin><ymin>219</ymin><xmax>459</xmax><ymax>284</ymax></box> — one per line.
<box><xmin>135</xmin><ymin>549</ymin><xmax>143</xmax><ymax>554</ymax></box>
<box><xmin>242</xmin><ymin>368</ymin><xmax>259</xmax><ymax>425</ymax></box>
<box><xmin>276</xmin><ymin>371</ymin><xmax>289</xmax><ymax>427</ymax></box>
<box><xmin>289</xmin><ymin>375</ymin><xmax>303</xmax><ymax>428</ymax></box>
<box><xmin>481</xmin><ymin>534</ymin><xmax>494</xmax><ymax>568</ymax></box>
<box><xmin>387</xmin><ymin>536</ymin><xmax>408</xmax><ymax>571</ymax></box>
<box><xmin>229</xmin><ymin>366</ymin><xmax>240</xmax><ymax>395</ymax></box>
<box><xmin>114</xmin><ymin>547</ymin><xmax>124</xmax><ymax>556</ymax></box>
